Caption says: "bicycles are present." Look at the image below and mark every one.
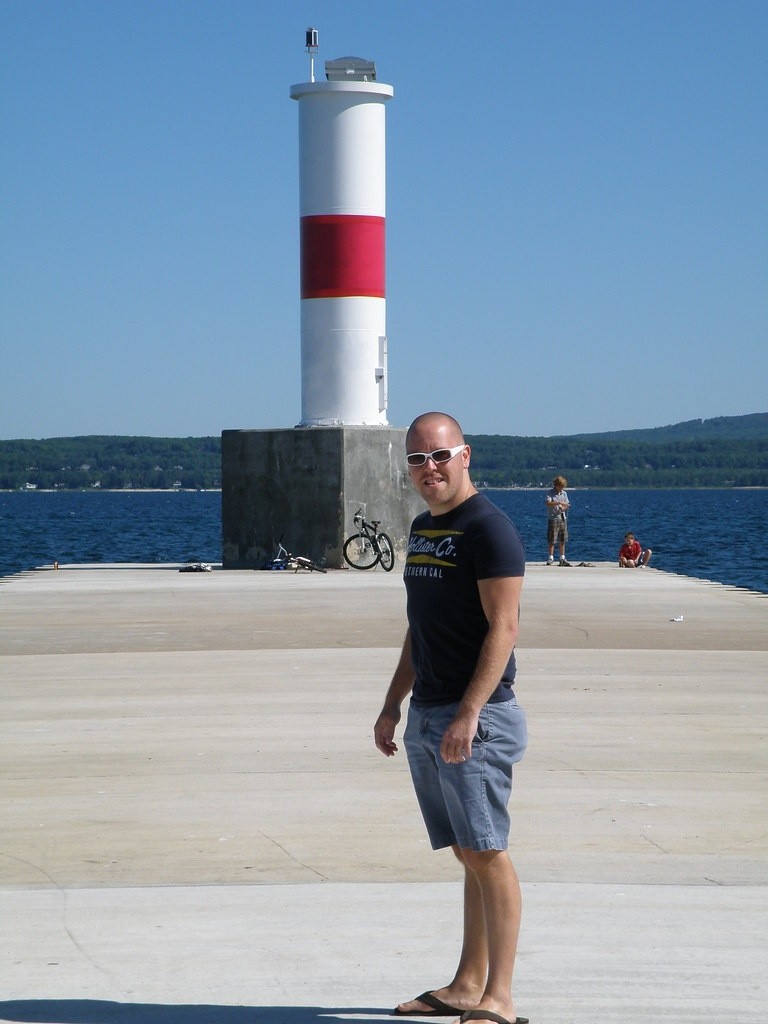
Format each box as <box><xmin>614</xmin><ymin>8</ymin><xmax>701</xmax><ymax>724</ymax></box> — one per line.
<box><xmin>343</xmin><ymin>508</ymin><xmax>394</xmax><ymax>571</ymax></box>
<box><xmin>268</xmin><ymin>534</ymin><xmax>327</xmax><ymax>574</ymax></box>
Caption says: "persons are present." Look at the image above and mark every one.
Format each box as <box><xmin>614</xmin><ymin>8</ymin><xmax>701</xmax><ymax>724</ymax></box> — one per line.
<box><xmin>372</xmin><ymin>410</ymin><xmax>531</xmax><ymax>1023</ymax></box>
<box><xmin>545</xmin><ymin>476</ymin><xmax>573</xmax><ymax>566</ymax></box>
<box><xmin>619</xmin><ymin>532</ymin><xmax>653</xmax><ymax>569</ymax></box>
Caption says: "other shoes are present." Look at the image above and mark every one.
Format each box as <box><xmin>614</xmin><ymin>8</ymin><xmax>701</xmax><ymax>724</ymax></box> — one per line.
<box><xmin>547</xmin><ymin>558</ymin><xmax>554</xmax><ymax>565</ymax></box>
<box><xmin>574</xmin><ymin>561</ymin><xmax>595</xmax><ymax>567</ymax></box>
<box><xmin>559</xmin><ymin>559</ymin><xmax>571</xmax><ymax>566</ymax></box>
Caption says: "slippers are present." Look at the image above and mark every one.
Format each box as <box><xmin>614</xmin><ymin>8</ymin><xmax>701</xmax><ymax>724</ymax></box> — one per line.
<box><xmin>459</xmin><ymin>1008</ymin><xmax>530</xmax><ymax>1024</ymax></box>
<box><xmin>393</xmin><ymin>989</ymin><xmax>466</xmax><ymax>1015</ymax></box>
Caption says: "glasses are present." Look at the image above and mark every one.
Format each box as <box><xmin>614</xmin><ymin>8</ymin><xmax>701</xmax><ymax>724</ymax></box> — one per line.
<box><xmin>406</xmin><ymin>445</ymin><xmax>466</xmax><ymax>466</ymax></box>
